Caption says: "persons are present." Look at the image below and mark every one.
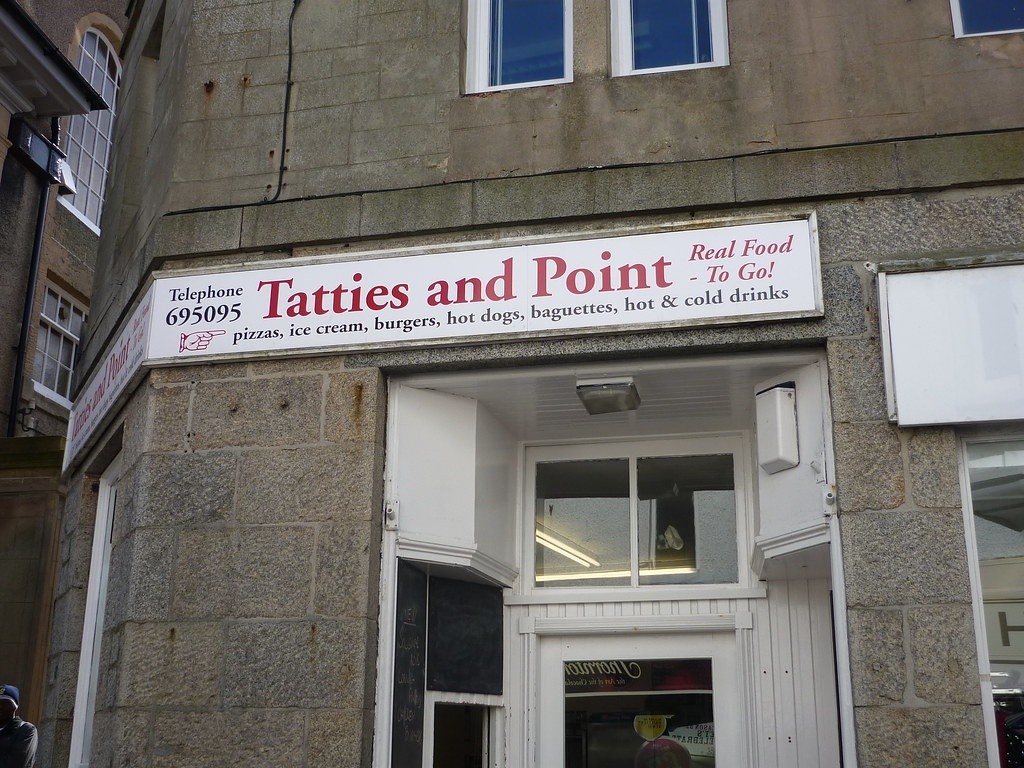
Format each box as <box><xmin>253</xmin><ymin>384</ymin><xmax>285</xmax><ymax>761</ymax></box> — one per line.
<box><xmin>0</xmin><ymin>684</ymin><xmax>38</xmax><ymax>768</ymax></box>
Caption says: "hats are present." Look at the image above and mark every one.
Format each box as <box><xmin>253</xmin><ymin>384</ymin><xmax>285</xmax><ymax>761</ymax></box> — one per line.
<box><xmin>0</xmin><ymin>684</ymin><xmax>20</xmax><ymax>710</ymax></box>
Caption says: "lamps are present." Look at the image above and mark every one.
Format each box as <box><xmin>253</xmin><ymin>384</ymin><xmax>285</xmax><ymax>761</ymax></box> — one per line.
<box><xmin>536</xmin><ymin>521</ymin><xmax>601</xmax><ymax>568</ymax></box>
<box><xmin>575</xmin><ymin>376</ymin><xmax>641</xmax><ymax>416</ymax></box>
